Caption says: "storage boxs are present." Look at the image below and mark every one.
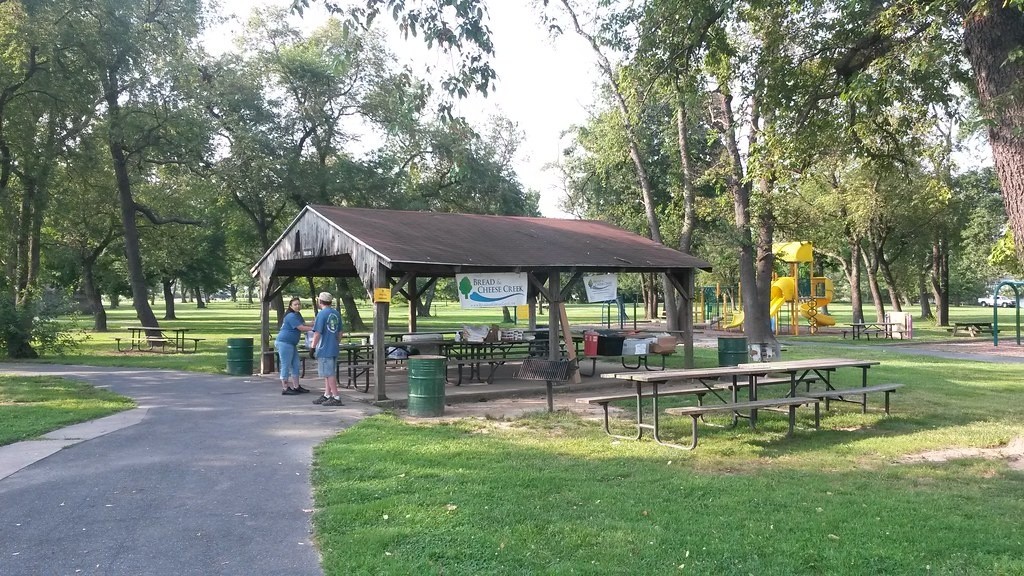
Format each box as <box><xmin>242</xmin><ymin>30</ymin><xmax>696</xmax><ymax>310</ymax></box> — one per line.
<box><xmin>597</xmin><ymin>334</ymin><xmax>677</xmax><ymax>356</ymax></box>
<box><xmin>486</xmin><ymin>324</ymin><xmax>499</xmax><ymax>342</ymax></box>
<box><xmin>305</xmin><ymin>331</ymin><xmax>313</xmax><ymax>348</ymax></box>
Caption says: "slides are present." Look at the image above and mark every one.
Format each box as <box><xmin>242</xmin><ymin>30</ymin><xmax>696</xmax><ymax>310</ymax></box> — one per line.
<box><xmin>770</xmin><ymin>296</ymin><xmax>785</xmax><ymax>318</ymax></box>
<box><xmin>723</xmin><ymin>310</ymin><xmax>745</xmax><ymax>329</ymax></box>
<box><xmin>800</xmin><ymin>298</ymin><xmax>836</xmax><ymax>325</ymax></box>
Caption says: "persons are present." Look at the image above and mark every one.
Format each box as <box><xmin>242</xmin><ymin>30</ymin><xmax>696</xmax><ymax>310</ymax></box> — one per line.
<box><xmin>275</xmin><ymin>298</ymin><xmax>313</xmax><ymax>395</ymax></box>
<box><xmin>309</xmin><ymin>292</ymin><xmax>343</xmax><ymax>406</ymax></box>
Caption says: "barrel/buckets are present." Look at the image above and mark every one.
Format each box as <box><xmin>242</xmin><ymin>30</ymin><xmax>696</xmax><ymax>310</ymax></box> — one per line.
<box><xmin>407</xmin><ymin>355</ymin><xmax>448</xmax><ymax>418</ymax></box>
<box><xmin>226</xmin><ymin>338</ymin><xmax>254</xmax><ymax>376</ymax></box>
<box><xmin>718</xmin><ymin>336</ymin><xmax>748</xmax><ymax>383</ymax></box>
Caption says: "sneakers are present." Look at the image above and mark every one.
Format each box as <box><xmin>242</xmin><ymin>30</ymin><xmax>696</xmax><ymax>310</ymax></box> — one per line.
<box><xmin>281</xmin><ymin>387</ymin><xmax>299</xmax><ymax>395</ymax></box>
<box><xmin>322</xmin><ymin>396</ymin><xmax>342</xmax><ymax>405</ymax></box>
<box><xmin>294</xmin><ymin>385</ymin><xmax>310</xmax><ymax>393</ymax></box>
<box><xmin>313</xmin><ymin>394</ymin><xmax>328</xmax><ymax>404</ymax></box>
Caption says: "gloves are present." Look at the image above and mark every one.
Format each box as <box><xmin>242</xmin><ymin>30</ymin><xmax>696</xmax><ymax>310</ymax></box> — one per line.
<box><xmin>309</xmin><ymin>348</ymin><xmax>316</xmax><ymax>360</ymax></box>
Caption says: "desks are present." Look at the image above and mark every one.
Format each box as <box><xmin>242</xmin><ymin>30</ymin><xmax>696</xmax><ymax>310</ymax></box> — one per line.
<box><xmin>952</xmin><ymin>322</ymin><xmax>991</xmax><ymax>337</ymax></box>
<box><xmin>128</xmin><ymin>327</ymin><xmax>196</xmax><ymax>354</ymax></box>
<box><xmin>337</xmin><ymin>330</ymin><xmax>494</xmax><ymax>393</ymax></box>
<box><xmin>844</xmin><ymin>323</ymin><xmax>895</xmax><ymax>340</ymax></box>
<box><xmin>600</xmin><ymin>358</ymin><xmax>880</xmax><ymax>451</ymax></box>
<box><xmin>561</xmin><ymin>334</ymin><xmax>665</xmax><ymax>377</ymax></box>
<box><xmin>505</xmin><ymin>339</ymin><xmax>552</xmax><ymax>344</ymax></box>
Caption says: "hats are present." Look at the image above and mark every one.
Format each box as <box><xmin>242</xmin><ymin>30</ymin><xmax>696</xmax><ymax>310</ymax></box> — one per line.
<box><xmin>316</xmin><ymin>292</ymin><xmax>333</xmax><ymax>303</ymax></box>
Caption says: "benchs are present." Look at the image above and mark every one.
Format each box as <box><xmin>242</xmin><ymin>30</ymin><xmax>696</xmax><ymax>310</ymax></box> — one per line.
<box><xmin>947</xmin><ymin>328</ymin><xmax>1000</xmax><ymax>333</ymax></box>
<box><xmin>264</xmin><ymin>349</ymin><xmax>310</xmax><ymax>378</ymax></box>
<box><xmin>109</xmin><ymin>336</ymin><xmax>206</xmax><ymax>342</ymax></box>
<box><xmin>841</xmin><ymin>329</ymin><xmax>903</xmax><ymax>340</ymax></box>
<box><xmin>559</xmin><ymin>349</ymin><xmax>677</xmax><ymax>358</ymax></box>
<box><xmin>337</xmin><ymin>344</ymin><xmax>549</xmax><ymax>368</ymax></box>
<box><xmin>576</xmin><ymin>375</ymin><xmax>907</xmax><ymax>416</ymax></box>
